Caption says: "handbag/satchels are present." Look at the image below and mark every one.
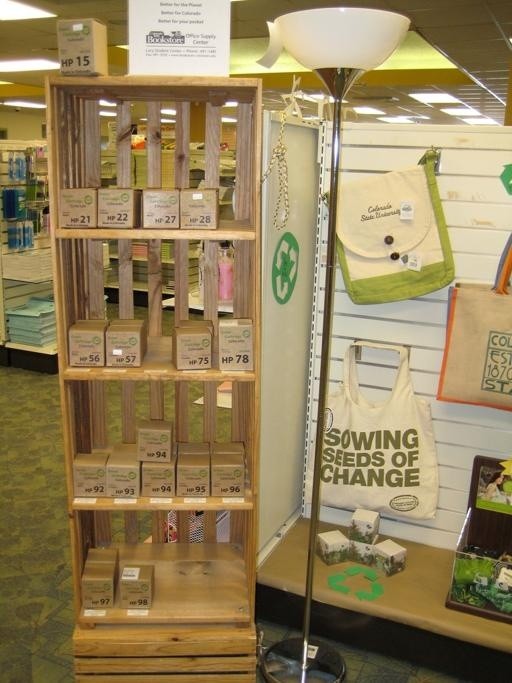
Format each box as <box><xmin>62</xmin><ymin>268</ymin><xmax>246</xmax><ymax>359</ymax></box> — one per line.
<box><xmin>437</xmin><ymin>232</ymin><xmax>512</xmax><ymax>411</ymax></box>
<box><xmin>336</xmin><ymin>150</ymin><xmax>454</xmax><ymax>306</ymax></box>
<box><xmin>319</xmin><ymin>342</ymin><xmax>438</xmax><ymax>519</ymax></box>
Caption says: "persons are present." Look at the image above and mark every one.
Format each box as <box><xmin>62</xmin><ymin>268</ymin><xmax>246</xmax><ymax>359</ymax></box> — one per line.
<box><xmin>480</xmin><ymin>471</ymin><xmax>509</xmax><ymax>505</ymax></box>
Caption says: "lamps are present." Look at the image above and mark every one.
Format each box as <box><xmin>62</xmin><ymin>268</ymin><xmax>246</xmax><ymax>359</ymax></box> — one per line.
<box><xmin>263</xmin><ymin>6</ymin><xmax>413</xmax><ymax>682</ymax></box>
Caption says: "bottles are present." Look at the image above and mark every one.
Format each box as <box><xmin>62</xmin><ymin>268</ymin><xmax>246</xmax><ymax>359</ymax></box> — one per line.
<box><xmin>198</xmin><ymin>241</ymin><xmax>205</xmax><ymax>303</ymax></box>
<box><xmin>218</xmin><ymin>241</ymin><xmax>235</xmax><ymax>301</ymax></box>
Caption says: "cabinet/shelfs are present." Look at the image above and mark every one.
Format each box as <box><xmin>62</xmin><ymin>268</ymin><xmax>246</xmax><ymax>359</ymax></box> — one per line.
<box><xmin>45</xmin><ymin>74</ymin><xmax>269</xmax><ymax>682</ymax></box>
